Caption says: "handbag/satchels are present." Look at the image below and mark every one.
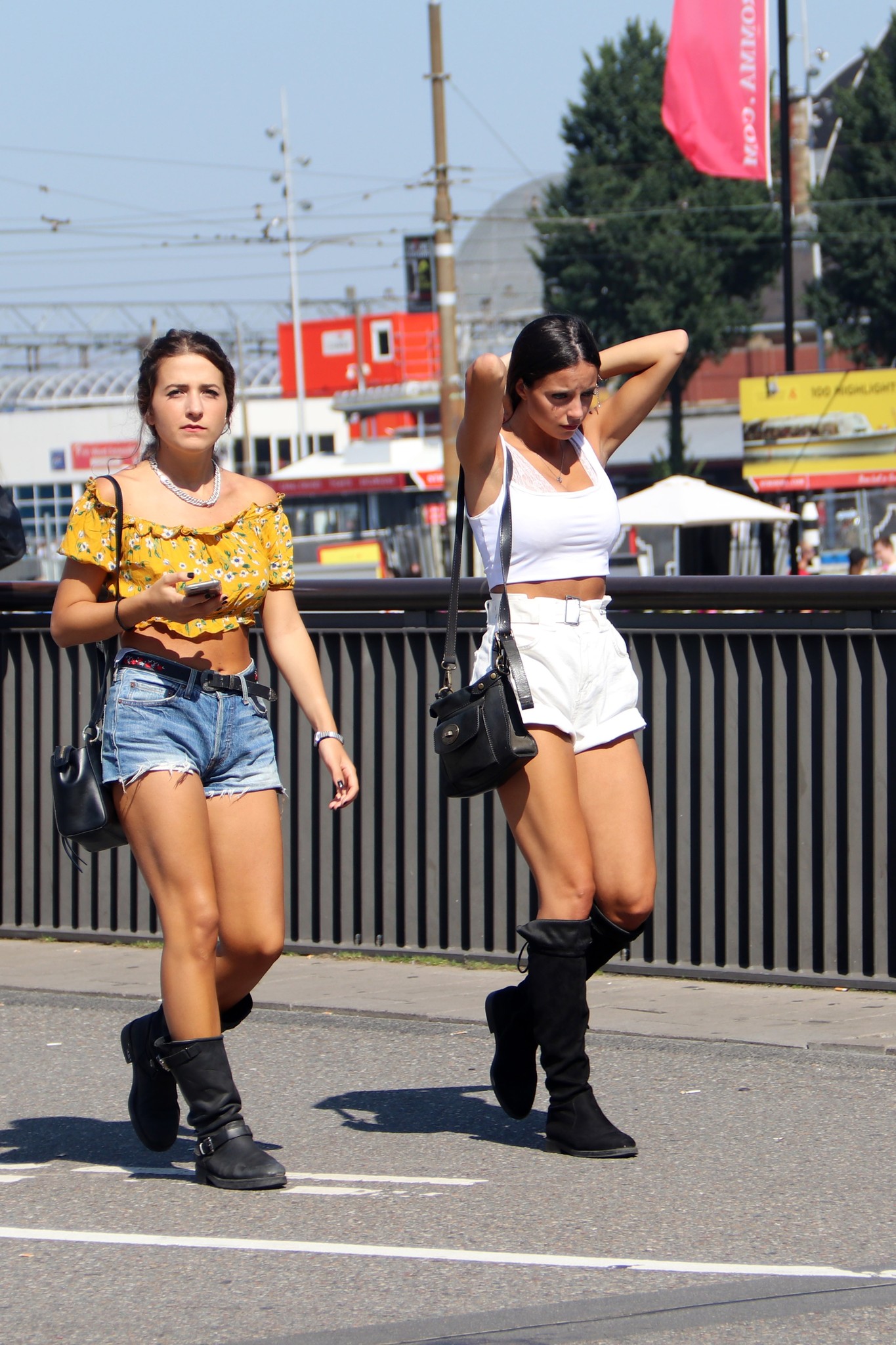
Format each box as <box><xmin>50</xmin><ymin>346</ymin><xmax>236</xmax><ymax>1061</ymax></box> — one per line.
<box><xmin>50</xmin><ymin>741</ymin><xmax>129</xmax><ymax>873</ymax></box>
<box><xmin>429</xmin><ymin>668</ymin><xmax>538</xmax><ymax>798</ymax></box>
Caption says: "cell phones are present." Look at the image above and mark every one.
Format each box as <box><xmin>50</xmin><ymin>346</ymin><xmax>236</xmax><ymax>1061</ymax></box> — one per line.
<box><xmin>184</xmin><ymin>580</ymin><xmax>223</xmax><ymax>599</ymax></box>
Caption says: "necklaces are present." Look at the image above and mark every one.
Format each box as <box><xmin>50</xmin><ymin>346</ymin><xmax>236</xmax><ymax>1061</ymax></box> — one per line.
<box><xmin>507</xmin><ymin>421</ymin><xmax>564</xmax><ymax>483</ymax></box>
<box><xmin>148</xmin><ymin>453</ymin><xmax>221</xmax><ymax>508</ymax></box>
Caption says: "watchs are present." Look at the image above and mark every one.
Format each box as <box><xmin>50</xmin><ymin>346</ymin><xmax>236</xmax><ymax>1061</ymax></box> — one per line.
<box><xmin>313</xmin><ymin>731</ymin><xmax>344</xmax><ymax>749</ymax></box>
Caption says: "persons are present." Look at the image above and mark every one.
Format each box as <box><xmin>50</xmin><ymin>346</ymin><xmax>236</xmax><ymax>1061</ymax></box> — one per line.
<box><xmin>50</xmin><ymin>327</ymin><xmax>361</xmax><ymax>1190</ymax></box>
<box><xmin>789</xmin><ymin>537</ymin><xmax>896</xmax><ymax>575</ymax></box>
<box><xmin>0</xmin><ymin>485</ymin><xmax>27</xmax><ymax>571</ymax></box>
<box><xmin>458</xmin><ymin>316</ymin><xmax>690</xmax><ymax>1160</ymax></box>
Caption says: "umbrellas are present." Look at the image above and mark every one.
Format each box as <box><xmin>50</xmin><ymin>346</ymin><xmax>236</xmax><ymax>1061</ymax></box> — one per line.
<box><xmin>617</xmin><ymin>474</ymin><xmax>801</xmax><ymax>527</ymax></box>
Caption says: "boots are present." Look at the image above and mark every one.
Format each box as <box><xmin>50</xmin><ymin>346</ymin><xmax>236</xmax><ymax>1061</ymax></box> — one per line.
<box><xmin>154</xmin><ymin>1034</ymin><xmax>287</xmax><ymax>1190</ymax></box>
<box><xmin>486</xmin><ymin>904</ymin><xmax>649</xmax><ymax>1120</ymax></box>
<box><xmin>119</xmin><ymin>993</ymin><xmax>253</xmax><ymax>1152</ymax></box>
<box><xmin>515</xmin><ymin>917</ymin><xmax>639</xmax><ymax>1158</ymax></box>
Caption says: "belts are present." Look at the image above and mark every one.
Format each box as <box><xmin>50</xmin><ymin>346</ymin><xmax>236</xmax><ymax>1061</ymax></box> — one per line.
<box><xmin>120</xmin><ymin>653</ymin><xmax>278</xmax><ymax>701</ymax></box>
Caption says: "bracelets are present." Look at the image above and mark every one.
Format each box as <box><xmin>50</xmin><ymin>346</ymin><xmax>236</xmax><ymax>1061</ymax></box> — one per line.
<box><xmin>115</xmin><ymin>597</ymin><xmax>136</xmax><ymax>632</ymax></box>
<box><xmin>593</xmin><ymin>374</ymin><xmax>609</xmax><ymax>408</ymax></box>
